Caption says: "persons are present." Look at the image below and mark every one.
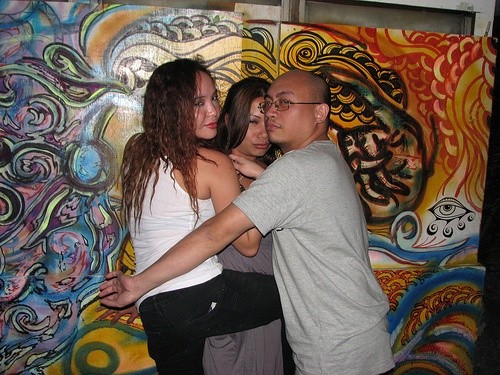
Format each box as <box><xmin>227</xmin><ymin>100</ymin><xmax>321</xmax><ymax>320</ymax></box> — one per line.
<box><xmin>201</xmin><ymin>75</ymin><xmax>284</xmax><ymax>375</ymax></box>
<box><xmin>116</xmin><ymin>58</ymin><xmax>296</xmax><ymax>375</ymax></box>
<box><xmin>99</xmin><ymin>70</ymin><xmax>397</xmax><ymax>375</ymax></box>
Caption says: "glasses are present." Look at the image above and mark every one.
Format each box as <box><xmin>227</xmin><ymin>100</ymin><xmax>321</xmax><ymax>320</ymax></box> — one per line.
<box><xmin>258</xmin><ymin>99</ymin><xmax>321</xmax><ymax>114</ymax></box>
